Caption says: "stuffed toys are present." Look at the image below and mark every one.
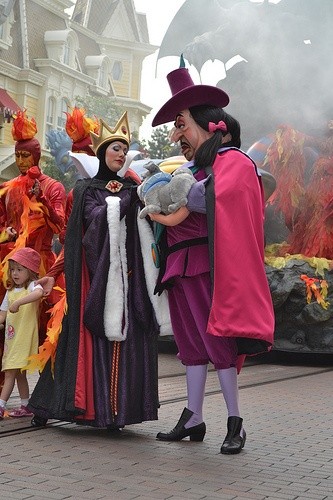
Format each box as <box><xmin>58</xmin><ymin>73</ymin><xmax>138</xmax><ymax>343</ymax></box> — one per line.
<box><xmin>137</xmin><ymin>160</ymin><xmax>198</xmax><ymax>218</ymax></box>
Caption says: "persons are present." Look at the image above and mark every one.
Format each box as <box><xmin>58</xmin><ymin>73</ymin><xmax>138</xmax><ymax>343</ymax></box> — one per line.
<box><xmin>0</xmin><ymin>139</ymin><xmax>142</xmax><ymax>392</ymax></box>
<box><xmin>26</xmin><ymin>114</ymin><xmax>161</xmax><ymax>435</ymax></box>
<box><xmin>148</xmin><ymin>67</ymin><xmax>274</xmax><ymax>454</ymax></box>
<box><xmin>0</xmin><ymin>248</ymin><xmax>44</xmax><ymax>420</ymax></box>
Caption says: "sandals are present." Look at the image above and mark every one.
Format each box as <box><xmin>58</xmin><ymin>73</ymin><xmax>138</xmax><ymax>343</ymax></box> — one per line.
<box><xmin>9</xmin><ymin>404</ymin><xmax>34</xmax><ymax>417</ymax></box>
<box><xmin>0</xmin><ymin>406</ymin><xmax>5</xmax><ymax>420</ymax></box>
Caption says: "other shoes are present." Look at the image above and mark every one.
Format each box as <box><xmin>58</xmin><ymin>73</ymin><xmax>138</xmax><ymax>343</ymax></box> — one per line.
<box><xmin>31</xmin><ymin>415</ymin><xmax>48</xmax><ymax>426</ymax></box>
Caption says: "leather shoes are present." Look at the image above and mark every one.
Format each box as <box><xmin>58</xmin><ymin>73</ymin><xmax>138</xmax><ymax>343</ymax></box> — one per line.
<box><xmin>220</xmin><ymin>416</ymin><xmax>246</xmax><ymax>454</ymax></box>
<box><xmin>156</xmin><ymin>407</ymin><xmax>206</xmax><ymax>441</ymax></box>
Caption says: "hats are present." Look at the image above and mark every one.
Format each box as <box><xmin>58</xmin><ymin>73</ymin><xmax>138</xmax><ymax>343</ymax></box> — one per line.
<box><xmin>8</xmin><ymin>247</ymin><xmax>42</xmax><ymax>275</ymax></box>
